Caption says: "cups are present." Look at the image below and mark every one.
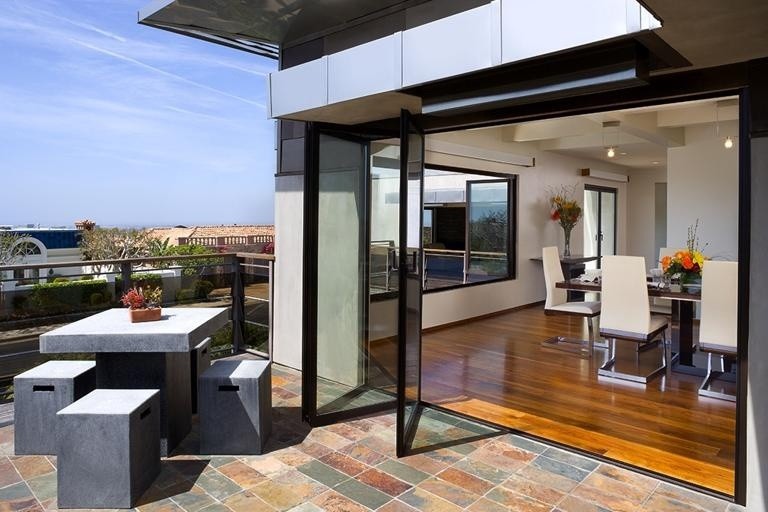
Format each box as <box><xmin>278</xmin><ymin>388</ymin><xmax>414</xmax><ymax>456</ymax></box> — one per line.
<box><xmin>649</xmin><ymin>268</ymin><xmax>661</xmax><ymax>278</ymax></box>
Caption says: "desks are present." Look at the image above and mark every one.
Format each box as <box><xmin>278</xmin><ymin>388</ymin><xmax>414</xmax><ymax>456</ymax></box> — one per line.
<box><xmin>39</xmin><ymin>307</ymin><xmax>229</xmax><ymax>458</ymax></box>
<box><xmin>529</xmin><ymin>256</ymin><xmax>603</xmax><ymax>302</ymax></box>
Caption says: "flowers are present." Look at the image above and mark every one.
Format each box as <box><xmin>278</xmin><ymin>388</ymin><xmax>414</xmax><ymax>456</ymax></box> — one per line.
<box><xmin>119</xmin><ymin>285</ymin><xmax>163</xmax><ymax>309</ymax></box>
<box><xmin>542</xmin><ymin>181</ymin><xmax>584</xmax><ymax>254</ymax></box>
<box><xmin>657</xmin><ymin>214</ymin><xmax>713</xmax><ymax>285</ymax></box>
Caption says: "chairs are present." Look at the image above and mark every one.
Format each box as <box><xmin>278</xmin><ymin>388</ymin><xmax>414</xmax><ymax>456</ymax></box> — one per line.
<box><xmin>597</xmin><ymin>255</ymin><xmax>668</xmax><ymax>384</ymax></box>
<box><xmin>698</xmin><ymin>260</ymin><xmax>738</xmax><ymax>404</ymax></box>
<box><xmin>540</xmin><ymin>246</ymin><xmax>610</xmax><ymax>360</ymax></box>
<box><xmin>636</xmin><ymin>247</ymin><xmax>697</xmax><ymax>353</ymax></box>
<box><xmin>427</xmin><ymin>243</ymin><xmax>447</xmax><ymax>254</ymax></box>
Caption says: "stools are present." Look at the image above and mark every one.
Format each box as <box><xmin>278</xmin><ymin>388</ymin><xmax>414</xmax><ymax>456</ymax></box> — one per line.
<box><xmin>199</xmin><ymin>358</ymin><xmax>272</xmax><ymax>455</ymax></box>
<box><xmin>191</xmin><ymin>336</ymin><xmax>211</xmax><ymax>414</ymax></box>
<box><xmin>55</xmin><ymin>388</ymin><xmax>160</xmax><ymax>509</ymax></box>
<box><xmin>13</xmin><ymin>360</ymin><xmax>97</xmax><ymax>456</ymax></box>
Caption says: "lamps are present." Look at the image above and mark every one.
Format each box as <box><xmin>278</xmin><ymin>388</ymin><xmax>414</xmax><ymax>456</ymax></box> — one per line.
<box><xmin>714</xmin><ymin>98</ymin><xmax>739</xmax><ymax>149</ymax></box>
<box><xmin>600</xmin><ymin>120</ymin><xmax>622</xmax><ymax>159</ymax></box>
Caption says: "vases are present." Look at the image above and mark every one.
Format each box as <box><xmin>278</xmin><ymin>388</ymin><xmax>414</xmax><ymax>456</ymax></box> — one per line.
<box><xmin>129</xmin><ymin>306</ymin><xmax>161</xmax><ymax>323</ymax></box>
<box><xmin>559</xmin><ymin>222</ymin><xmax>574</xmax><ymax>258</ymax></box>
<box><xmin>679</xmin><ymin>272</ymin><xmax>702</xmax><ymax>295</ymax></box>
